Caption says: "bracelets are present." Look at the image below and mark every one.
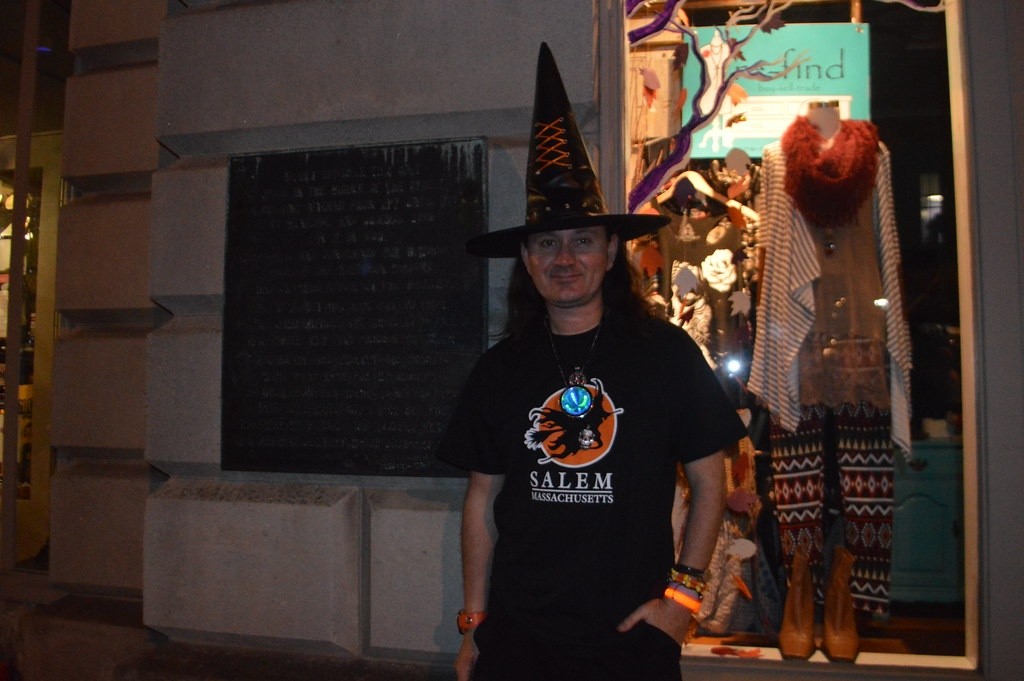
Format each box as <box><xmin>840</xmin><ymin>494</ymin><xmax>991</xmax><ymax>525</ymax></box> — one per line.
<box><xmin>457</xmin><ymin>611</ymin><xmax>486</xmax><ymax>634</ymax></box>
<box><xmin>665</xmin><ymin>564</ymin><xmax>707</xmax><ymax>614</ymax></box>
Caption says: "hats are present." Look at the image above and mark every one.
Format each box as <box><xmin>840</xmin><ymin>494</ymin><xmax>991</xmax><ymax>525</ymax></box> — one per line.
<box><xmin>466</xmin><ymin>41</ymin><xmax>672</xmax><ymax>258</ymax></box>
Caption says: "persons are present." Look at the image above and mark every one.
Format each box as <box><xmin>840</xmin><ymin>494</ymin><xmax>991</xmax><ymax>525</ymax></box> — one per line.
<box><xmin>749</xmin><ymin>103</ymin><xmax>913</xmax><ymax>662</ymax></box>
<box><xmin>455</xmin><ymin>226</ymin><xmax>749</xmax><ymax>681</ymax></box>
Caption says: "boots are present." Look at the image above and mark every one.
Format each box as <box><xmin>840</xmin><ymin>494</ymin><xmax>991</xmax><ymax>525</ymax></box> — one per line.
<box><xmin>823</xmin><ymin>545</ymin><xmax>861</xmax><ymax>663</ymax></box>
<box><xmin>779</xmin><ymin>548</ymin><xmax>816</xmax><ymax>661</ymax></box>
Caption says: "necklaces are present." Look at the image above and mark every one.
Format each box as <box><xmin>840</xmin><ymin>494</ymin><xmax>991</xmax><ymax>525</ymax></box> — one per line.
<box><xmin>544</xmin><ymin>314</ymin><xmax>604</xmax><ymax>386</ymax></box>
